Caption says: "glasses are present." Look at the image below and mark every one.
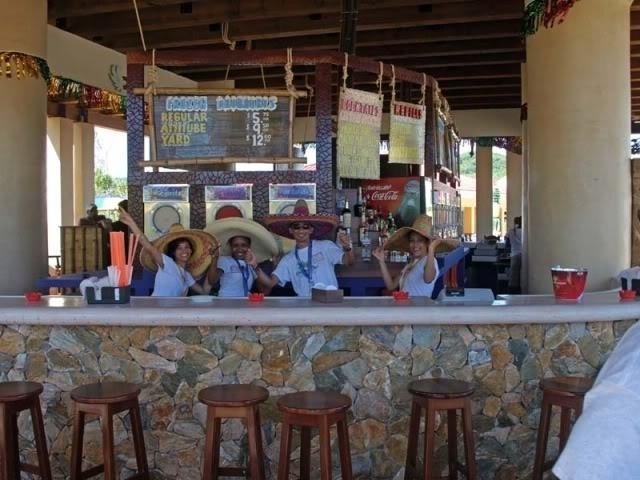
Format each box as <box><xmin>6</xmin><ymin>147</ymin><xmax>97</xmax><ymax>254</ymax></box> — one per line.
<box><xmin>292</xmin><ymin>223</ymin><xmax>311</xmax><ymax>230</ymax></box>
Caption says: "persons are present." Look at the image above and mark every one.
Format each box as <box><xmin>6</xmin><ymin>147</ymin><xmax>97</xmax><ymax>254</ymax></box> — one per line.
<box><xmin>504</xmin><ymin>217</ymin><xmax>522</xmax><ymax>291</ymax></box>
<box><xmin>373</xmin><ymin>215</ymin><xmax>458</xmax><ymax>299</ymax></box>
<box><xmin>118</xmin><ymin>206</ymin><xmax>212</xmax><ymax>298</ymax></box>
<box><xmin>78</xmin><ymin>202</ymin><xmax>105</xmax><ymax>226</ymax></box>
<box><xmin>244</xmin><ymin>200</ymin><xmax>351</xmax><ymax>299</ymax></box>
<box><xmin>209</xmin><ymin>236</ymin><xmax>270</xmax><ymax>298</ymax></box>
<box><xmin>111</xmin><ymin>200</ymin><xmax>128</xmax><ymax>265</ymax></box>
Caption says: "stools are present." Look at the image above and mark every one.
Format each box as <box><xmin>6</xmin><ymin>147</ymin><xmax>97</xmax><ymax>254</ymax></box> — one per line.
<box><xmin>534</xmin><ymin>376</ymin><xmax>595</xmax><ymax>480</ymax></box>
<box><xmin>276</xmin><ymin>391</ymin><xmax>352</xmax><ymax>480</ymax></box>
<box><xmin>197</xmin><ymin>384</ymin><xmax>269</xmax><ymax>480</ymax></box>
<box><xmin>404</xmin><ymin>378</ymin><xmax>476</xmax><ymax>480</ymax></box>
<box><xmin>0</xmin><ymin>381</ymin><xmax>51</xmax><ymax>480</ymax></box>
<box><xmin>70</xmin><ymin>382</ymin><xmax>149</xmax><ymax>480</ymax></box>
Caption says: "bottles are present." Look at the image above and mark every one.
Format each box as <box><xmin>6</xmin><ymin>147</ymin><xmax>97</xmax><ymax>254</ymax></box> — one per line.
<box><xmin>355</xmin><ymin>186</ymin><xmax>396</xmax><ymax>262</ymax></box>
<box><xmin>397</xmin><ymin>181</ymin><xmax>420</xmax><ymax>228</ymax></box>
<box><xmin>334</xmin><ymin>199</ymin><xmax>353</xmax><ymax>259</ymax></box>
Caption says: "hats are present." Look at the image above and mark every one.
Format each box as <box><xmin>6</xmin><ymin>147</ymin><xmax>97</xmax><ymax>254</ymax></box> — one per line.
<box><xmin>383</xmin><ymin>213</ymin><xmax>461</xmax><ymax>254</ymax></box>
<box><xmin>202</xmin><ymin>215</ymin><xmax>279</xmax><ymax>265</ymax></box>
<box><xmin>86</xmin><ymin>204</ymin><xmax>97</xmax><ymax>214</ymax></box>
<box><xmin>139</xmin><ymin>222</ymin><xmax>221</xmax><ymax>282</ymax></box>
<box><xmin>262</xmin><ymin>198</ymin><xmax>341</xmax><ymax>241</ymax></box>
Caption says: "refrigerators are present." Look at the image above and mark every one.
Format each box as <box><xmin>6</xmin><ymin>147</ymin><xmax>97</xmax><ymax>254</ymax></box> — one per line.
<box><xmin>358</xmin><ymin>176</ymin><xmax>432</xmax><ymax>232</ymax></box>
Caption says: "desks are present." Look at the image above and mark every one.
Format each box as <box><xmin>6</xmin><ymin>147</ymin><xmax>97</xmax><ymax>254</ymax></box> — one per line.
<box><xmin>38</xmin><ymin>245</ymin><xmax>470</xmax><ymax>296</ymax></box>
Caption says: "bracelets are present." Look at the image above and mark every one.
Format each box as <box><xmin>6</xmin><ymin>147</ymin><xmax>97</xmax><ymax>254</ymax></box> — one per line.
<box><xmin>343</xmin><ymin>247</ymin><xmax>351</xmax><ymax>253</ymax></box>
<box><xmin>255</xmin><ymin>264</ymin><xmax>261</xmax><ymax>273</ymax></box>
